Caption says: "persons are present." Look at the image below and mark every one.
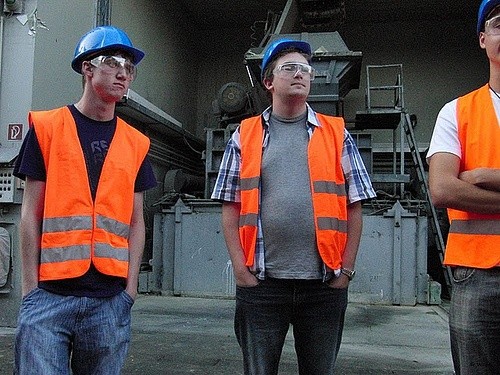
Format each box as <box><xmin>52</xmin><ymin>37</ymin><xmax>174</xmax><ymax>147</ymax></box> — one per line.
<box><xmin>12</xmin><ymin>26</ymin><xmax>158</xmax><ymax>375</ymax></box>
<box><xmin>211</xmin><ymin>37</ymin><xmax>377</xmax><ymax>375</ymax></box>
<box><xmin>426</xmin><ymin>0</ymin><xmax>500</xmax><ymax>375</ymax></box>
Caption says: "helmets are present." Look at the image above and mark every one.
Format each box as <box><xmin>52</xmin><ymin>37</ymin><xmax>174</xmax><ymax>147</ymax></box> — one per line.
<box><xmin>476</xmin><ymin>0</ymin><xmax>500</xmax><ymax>37</ymax></box>
<box><xmin>70</xmin><ymin>25</ymin><xmax>146</xmax><ymax>76</ymax></box>
<box><xmin>262</xmin><ymin>37</ymin><xmax>311</xmax><ymax>83</ymax></box>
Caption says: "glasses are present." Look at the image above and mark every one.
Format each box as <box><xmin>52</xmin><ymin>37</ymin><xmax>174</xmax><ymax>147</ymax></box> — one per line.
<box><xmin>484</xmin><ymin>16</ymin><xmax>500</xmax><ymax>35</ymax></box>
<box><xmin>89</xmin><ymin>56</ymin><xmax>138</xmax><ymax>82</ymax></box>
<box><xmin>266</xmin><ymin>62</ymin><xmax>316</xmax><ymax>79</ymax></box>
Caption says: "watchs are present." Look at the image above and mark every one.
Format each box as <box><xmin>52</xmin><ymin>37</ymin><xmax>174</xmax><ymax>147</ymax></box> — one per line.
<box><xmin>341</xmin><ymin>268</ymin><xmax>356</xmax><ymax>281</ymax></box>
